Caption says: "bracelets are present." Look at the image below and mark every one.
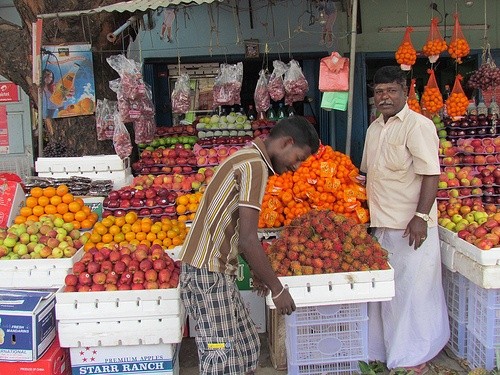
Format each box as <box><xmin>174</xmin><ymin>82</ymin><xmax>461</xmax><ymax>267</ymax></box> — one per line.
<box><xmin>271</xmin><ymin>285</ymin><xmax>285</xmax><ymax>299</ymax></box>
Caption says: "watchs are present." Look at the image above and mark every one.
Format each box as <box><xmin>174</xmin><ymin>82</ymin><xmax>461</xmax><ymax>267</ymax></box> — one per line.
<box><xmin>414</xmin><ymin>211</ymin><xmax>430</xmax><ymax>222</ymax></box>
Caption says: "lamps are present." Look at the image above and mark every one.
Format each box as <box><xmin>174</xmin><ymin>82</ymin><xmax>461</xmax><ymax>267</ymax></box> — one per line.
<box><xmin>378</xmin><ymin>24</ymin><xmax>490</xmax><ymax>32</ymax></box>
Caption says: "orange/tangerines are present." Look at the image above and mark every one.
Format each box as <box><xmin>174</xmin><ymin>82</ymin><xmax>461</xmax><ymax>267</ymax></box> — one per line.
<box><xmin>423</xmin><ymin>38</ymin><xmax>469</xmax><ymax>59</ymax></box>
<box><xmin>83</xmin><ymin>194</ymin><xmax>203</xmax><ymax>252</ymax></box>
<box><xmin>395</xmin><ymin>42</ymin><xmax>416</xmax><ymax>65</ymax></box>
<box><xmin>14</xmin><ymin>184</ymin><xmax>98</xmax><ymax>230</ymax></box>
<box><xmin>259</xmin><ymin>145</ymin><xmax>370</xmax><ymax>228</ymax></box>
<box><xmin>408</xmin><ymin>87</ymin><xmax>468</xmax><ymax>117</ymax></box>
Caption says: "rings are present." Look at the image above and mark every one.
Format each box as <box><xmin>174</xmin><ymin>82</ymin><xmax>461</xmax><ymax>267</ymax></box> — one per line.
<box><xmin>422</xmin><ymin>237</ymin><xmax>426</xmax><ymax>240</ymax></box>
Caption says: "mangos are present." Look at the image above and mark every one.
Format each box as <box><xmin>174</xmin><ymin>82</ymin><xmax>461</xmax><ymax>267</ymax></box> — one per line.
<box><xmin>59</xmin><ymin>98</ymin><xmax>95</xmax><ymax>116</ymax></box>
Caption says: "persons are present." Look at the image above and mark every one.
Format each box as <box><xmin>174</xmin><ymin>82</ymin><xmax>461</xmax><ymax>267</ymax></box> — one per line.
<box><xmin>359</xmin><ymin>65</ymin><xmax>451</xmax><ymax>375</ymax></box>
<box><xmin>177</xmin><ymin>115</ymin><xmax>321</xmax><ymax>375</ymax></box>
<box><xmin>42</xmin><ymin>69</ymin><xmax>74</xmax><ymax>118</ymax></box>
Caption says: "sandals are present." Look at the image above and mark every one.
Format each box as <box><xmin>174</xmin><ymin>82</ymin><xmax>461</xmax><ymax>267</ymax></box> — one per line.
<box><xmin>390</xmin><ymin>360</ymin><xmax>430</xmax><ymax>375</ymax></box>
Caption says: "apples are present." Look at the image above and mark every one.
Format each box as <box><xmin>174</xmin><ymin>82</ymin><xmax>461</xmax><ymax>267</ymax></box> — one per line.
<box><xmin>101</xmin><ymin>112</ymin><xmax>276</xmax><ymax>219</ymax></box>
<box><xmin>434</xmin><ymin>114</ymin><xmax>500</xmax><ymax>249</ymax></box>
<box><xmin>65</xmin><ymin>243</ymin><xmax>180</xmax><ymax>293</ymax></box>
<box><xmin>0</xmin><ymin>216</ymin><xmax>88</xmax><ymax>261</ymax></box>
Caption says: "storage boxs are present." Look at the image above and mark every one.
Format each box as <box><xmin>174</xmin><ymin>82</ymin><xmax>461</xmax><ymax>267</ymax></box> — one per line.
<box><xmin>189</xmin><ymin>254</ymin><xmax>396</xmax><ymax>375</ymax></box>
<box><xmin>438</xmin><ymin>225</ymin><xmax>500</xmax><ymax>375</ymax></box>
<box><xmin>35</xmin><ymin>155</ymin><xmax>133</xmax><ymax>191</ymax></box>
<box><xmin>0</xmin><ymin>180</ymin><xmax>192</xmax><ymax>375</ymax></box>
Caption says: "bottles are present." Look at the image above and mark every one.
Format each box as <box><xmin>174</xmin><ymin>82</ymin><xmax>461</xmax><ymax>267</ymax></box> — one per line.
<box><xmin>230</xmin><ymin>108</ymin><xmax>235</xmax><ymax>113</ymax></box>
<box><xmin>477</xmin><ymin>98</ymin><xmax>488</xmax><ymax>118</ymax></box>
<box><xmin>489</xmin><ymin>97</ymin><xmax>500</xmax><ymax>119</ymax></box>
<box><xmin>442</xmin><ymin>84</ymin><xmax>451</xmax><ymax>120</ymax></box>
<box><xmin>466</xmin><ymin>100</ymin><xmax>478</xmax><ymax>116</ymax></box>
<box><xmin>276</xmin><ymin>104</ymin><xmax>286</xmax><ymax>119</ymax></box>
<box><xmin>414</xmin><ymin>83</ymin><xmax>420</xmax><ymax>102</ymax></box>
<box><xmin>266</xmin><ymin>104</ymin><xmax>275</xmax><ymax>118</ymax></box>
<box><xmin>222</xmin><ymin>108</ymin><xmax>227</xmax><ymax>116</ymax></box>
<box><xmin>287</xmin><ymin>103</ymin><xmax>296</xmax><ymax>120</ymax></box>
<box><xmin>240</xmin><ymin>108</ymin><xmax>246</xmax><ymax>116</ymax></box>
<box><xmin>256</xmin><ymin>111</ymin><xmax>266</xmax><ymax>120</ymax></box>
<box><xmin>248</xmin><ymin>104</ymin><xmax>255</xmax><ymax>121</ymax></box>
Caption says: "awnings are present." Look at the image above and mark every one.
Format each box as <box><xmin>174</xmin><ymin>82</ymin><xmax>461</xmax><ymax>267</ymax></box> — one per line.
<box><xmin>37</xmin><ymin>0</ymin><xmax>224</xmax><ymax>19</ymax></box>
